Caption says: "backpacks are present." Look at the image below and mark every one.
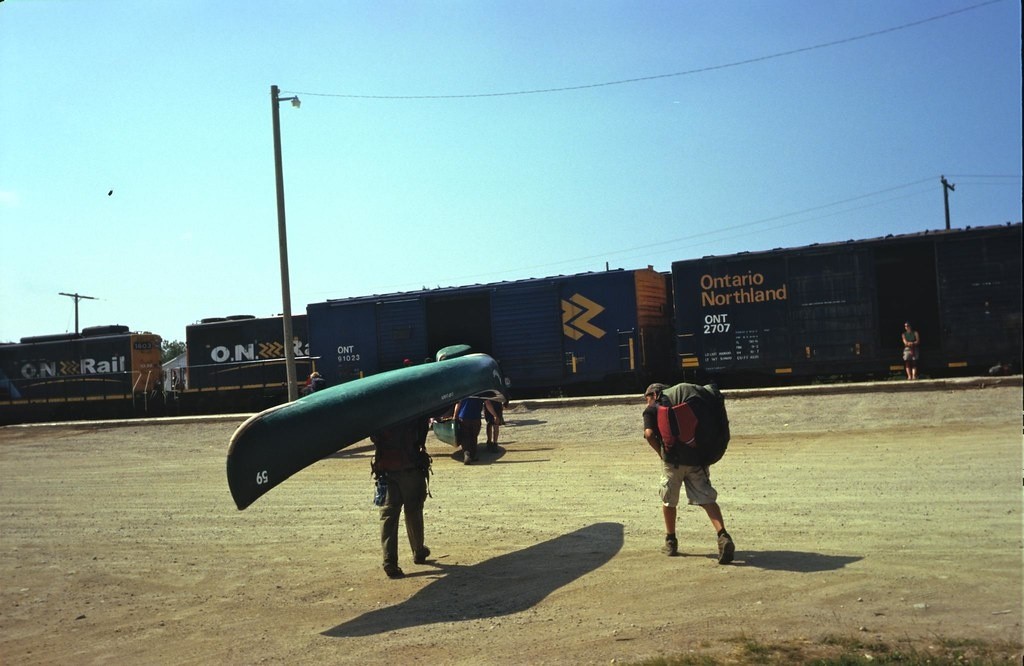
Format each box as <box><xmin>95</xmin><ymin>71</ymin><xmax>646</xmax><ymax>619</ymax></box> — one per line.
<box><xmin>654</xmin><ymin>382</ymin><xmax>731</xmax><ymax>466</ymax></box>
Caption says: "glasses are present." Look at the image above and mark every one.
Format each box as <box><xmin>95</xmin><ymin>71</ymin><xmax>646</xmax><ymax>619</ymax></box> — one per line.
<box><xmin>904</xmin><ymin>325</ymin><xmax>910</xmax><ymax>327</ymax></box>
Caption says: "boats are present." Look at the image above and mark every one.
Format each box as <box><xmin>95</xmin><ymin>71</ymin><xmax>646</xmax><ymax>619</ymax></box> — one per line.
<box><xmin>436</xmin><ymin>345</ymin><xmax>489</xmax><ymax>364</ymax></box>
<box><xmin>225</xmin><ymin>352</ymin><xmax>509</xmax><ymax>512</ymax></box>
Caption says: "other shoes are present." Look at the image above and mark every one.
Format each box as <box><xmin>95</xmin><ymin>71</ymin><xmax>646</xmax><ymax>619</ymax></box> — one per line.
<box><xmin>907</xmin><ymin>374</ymin><xmax>911</xmax><ymax>380</ymax></box>
<box><xmin>491</xmin><ymin>443</ymin><xmax>499</xmax><ymax>453</ymax></box>
<box><xmin>486</xmin><ymin>440</ymin><xmax>491</xmax><ymax>450</ymax></box>
<box><xmin>912</xmin><ymin>374</ymin><xmax>917</xmax><ymax>380</ymax></box>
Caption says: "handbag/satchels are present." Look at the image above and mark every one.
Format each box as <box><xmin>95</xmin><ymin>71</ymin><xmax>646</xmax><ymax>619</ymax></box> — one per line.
<box><xmin>412</xmin><ymin>437</ymin><xmax>434</xmax><ymax>486</ymax></box>
<box><xmin>311</xmin><ymin>378</ymin><xmax>326</xmax><ymax>393</ymax></box>
<box><xmin>908</xmin><ymin>343</ymin><xmax>916</xmax><ymax>357</ymax></box>
<box><xmin>373</xmin><ymin>482</ymin><xmax>389</xmax><ymax>506</ymax></box>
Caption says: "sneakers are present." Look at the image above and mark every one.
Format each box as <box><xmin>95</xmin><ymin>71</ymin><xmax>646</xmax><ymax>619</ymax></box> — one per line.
<box><xmin>661</xmin><ymin>538</ymin><xmax>678</xmax><ymax>556</ymax></box>
<box><xmin>382</xmin><ymin>562</ymin><xmax>406</xmax><ymax>580</ymax></box>
<box><xmin>413</xmin><ymin>544</ymin><xmax>431</xmax><ymax>565</ymax></box>
<box><xmin>716</xmin><ymin>532</ymin><xmax>735</xmax><ymax>565</ymax></box>
<box><xmin>463</xmin><ymin>451</ymin><xmax>472</xmax><ymax>465</ymax></box>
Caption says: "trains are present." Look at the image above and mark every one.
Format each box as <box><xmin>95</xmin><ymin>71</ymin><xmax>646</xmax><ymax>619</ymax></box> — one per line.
<box><xmin>0</xmin><ymin>224</ymin><xmax>1024</xmax><ymax>425</ymax></box>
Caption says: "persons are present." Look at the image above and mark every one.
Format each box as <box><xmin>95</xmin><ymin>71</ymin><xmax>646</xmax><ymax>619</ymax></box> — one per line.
<box><xmin>369</xmin><ymin>414</ymin><xmax>432</xmax><ymax>579</ymax></box>
<box><xmin>643</xmin><ymin>383</ymin><xmax>735</xmax><ymax>564</ymax></box>
<box><xmin>902</xmin><ymin>321</ymin><xmax>919</xmax><ymax>380</ymax></box>
<box><xmin>307</xmin><ymin>372</ymin><xmax>328</xmax><ymax>393</ymax></box>
<box><xmin>452</xmin><ymin>397</ymin><xmax>482</xmax><ymax>465</ymax></box>
<box><xmin>403</xmin><ymin>357</ymin><xmax>433</xmax><ymax>367</ymax></box>
<box><xmin>485</xmin><ymin>399</ymin><xmax>505</xmax><ymax>451</ymax></box>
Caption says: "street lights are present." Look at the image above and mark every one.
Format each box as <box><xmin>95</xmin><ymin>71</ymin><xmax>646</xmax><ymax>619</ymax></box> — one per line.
<box><xmin>269</xmin><ymin>84</ymin><xmax>303</xmax><ymax>404</ymax></box>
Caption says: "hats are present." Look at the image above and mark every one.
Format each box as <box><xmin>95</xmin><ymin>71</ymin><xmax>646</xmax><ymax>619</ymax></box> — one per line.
<box><xmin>310</xmin><ymin>372</ymin><xmax>320</xmax><ymax>379</ymax></box>
<box><xmin>644</xmin><ymin>382</ymin><xmax>666</xmax><ymax>399</ymax></box>
<box><xmin>403</xmin><ymin>358</ymin><xmax>410</xmax><ymax>364</ymax></box>
